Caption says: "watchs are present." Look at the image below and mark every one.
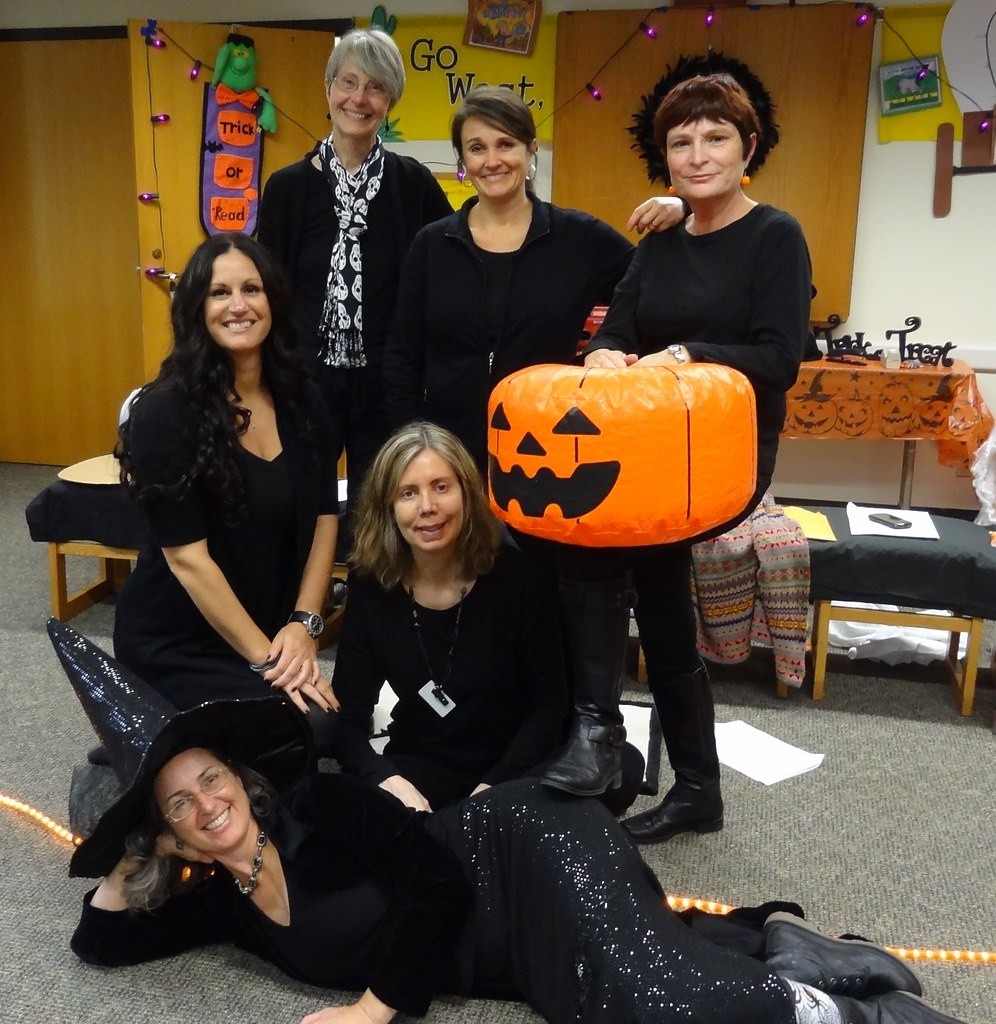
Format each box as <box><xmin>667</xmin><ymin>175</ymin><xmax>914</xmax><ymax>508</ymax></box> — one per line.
<box><xmin>667</xmin><ymin>345</ymin><xmax>685</xmax><ymax>365</ymax></box>
<box><xmin>287</xmin><ymin>611</ymin><xmax>324</xmax><ymax>637</ymax></box>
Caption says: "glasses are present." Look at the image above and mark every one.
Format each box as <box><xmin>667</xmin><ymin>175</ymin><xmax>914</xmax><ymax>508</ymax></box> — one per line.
<box><xmin>332</xmin><ymin>72</ymin><xmax>385</xmax><ymax>94</ymax></box>
<box><xmin>162</xmin><ymin>763</ymin><xmax>232</xmax><ymax>825</ymax></box>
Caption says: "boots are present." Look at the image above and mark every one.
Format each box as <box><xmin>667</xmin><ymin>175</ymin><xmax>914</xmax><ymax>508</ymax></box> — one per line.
<box><xmin>828</xmin><ymin>990</ymin><xmax>968</xmax><ymax>1024</ymax></box>
<box><xmin>619</xmin><ymin>657</ymin><xmax>724</xmax><ymax>846</ymax></box>
<box><xmin>539</xmin><ymin>586</ymin><xmax>636</xmax><ymax>797</ymax></box>
<box><xmin>761</xmin><ymin>912</ymin><xmax>923</xmax><ymax>1001</ymax></box>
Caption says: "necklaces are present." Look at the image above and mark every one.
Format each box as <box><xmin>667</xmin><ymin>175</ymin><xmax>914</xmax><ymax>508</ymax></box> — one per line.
<box><xmin>233</xmin><ymin>831</ymin><xmax>264</xmax><ymax>895</ymax></box>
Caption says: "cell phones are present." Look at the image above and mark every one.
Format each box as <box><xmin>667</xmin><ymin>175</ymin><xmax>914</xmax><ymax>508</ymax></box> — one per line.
<box><xmin>869</xmin><ymin>514</ymin><xmax>911</xmax><ymax>530</ymax></box>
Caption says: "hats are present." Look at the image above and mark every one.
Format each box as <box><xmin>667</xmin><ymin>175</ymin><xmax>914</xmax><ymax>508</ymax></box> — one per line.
<box><xmin>43</xmin><ymin>614</ymin><xmax>321</xmax><ymax>878</ymax></box>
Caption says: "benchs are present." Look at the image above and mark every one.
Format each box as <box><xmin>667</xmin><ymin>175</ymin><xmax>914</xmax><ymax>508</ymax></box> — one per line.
<box><xmin>25</xmin><ymin>452</ymin><xmax>996</xmax><ymax>716</ymax></box>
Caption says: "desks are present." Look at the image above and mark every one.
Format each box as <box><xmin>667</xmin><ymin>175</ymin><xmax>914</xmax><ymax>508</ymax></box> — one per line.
<box><xmin>779</xmin><ymin>350</ymin><xmax>995</xmax><ymax>510</ymax></box>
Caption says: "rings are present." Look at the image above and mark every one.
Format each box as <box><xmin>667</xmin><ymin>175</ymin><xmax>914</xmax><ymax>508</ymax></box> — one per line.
<box><xmin>652</xmin><ymin>221</ymin><xmax>659</xmax><ymax>227</ymax></box>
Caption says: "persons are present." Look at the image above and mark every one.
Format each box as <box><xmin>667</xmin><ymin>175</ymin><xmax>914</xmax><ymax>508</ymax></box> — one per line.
<box><xmin>47</xmin><ymin>618</ymin><xmax>976</xmax><ymax>1024</ymax></box>
<box><xmin>108</xmin><ymin>25</ymin><xmax>814</xmax><ymax>844</ymax></box>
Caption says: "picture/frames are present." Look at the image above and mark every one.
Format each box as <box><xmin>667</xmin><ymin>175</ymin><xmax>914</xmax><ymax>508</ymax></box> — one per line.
<box><xmin>879</xmin><ymin>55</ymin><xmax>942</xmax><ymax>118</ymax></box>
<box><xmin>462</xmin><ymin>0</ymin><xmax>543</xmax><ymax>56</ymax></box>
